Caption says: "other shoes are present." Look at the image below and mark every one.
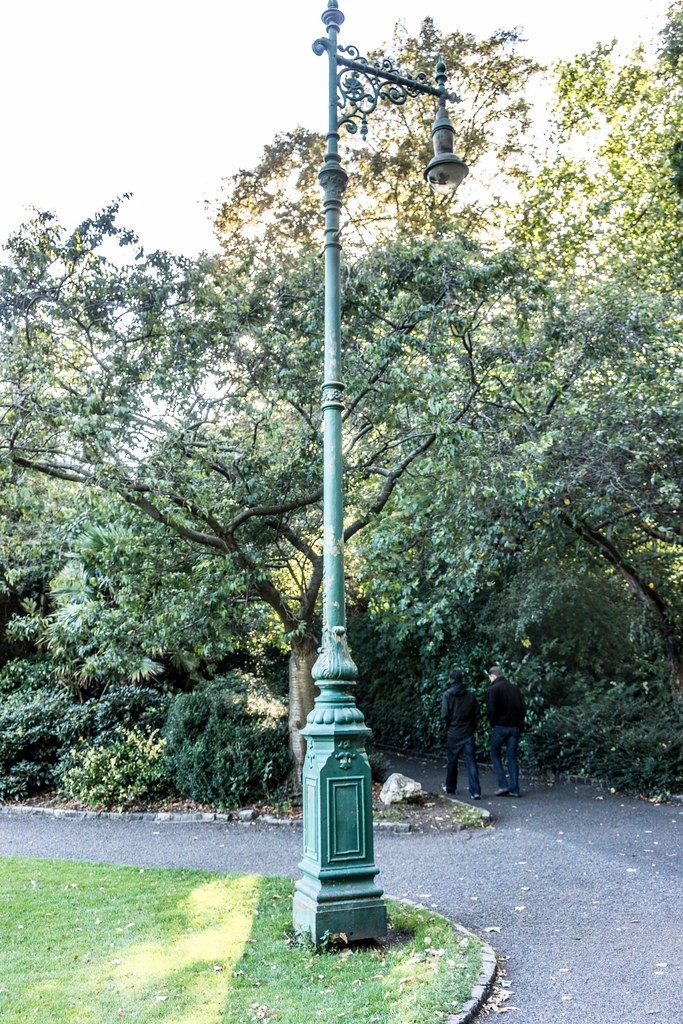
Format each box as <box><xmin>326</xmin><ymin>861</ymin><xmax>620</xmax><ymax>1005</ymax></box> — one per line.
<box><xmin>495</xmin><ymin>788</ymin><xmax>508</xmax><ymax>795</ymax></box>
<box><xmin>509</xmin><ymin>792</ymin><xmax>519</xmax><ymax>797</ymax></box>
<box><xmin>442</xmin><ymin>783</ymin><xmax>446</xmax><ymax>791</ymax></box>
<box><xmin>471</xmin><ymin>794</ymin><xmax>481</xmax><ymax>800</ymax></box>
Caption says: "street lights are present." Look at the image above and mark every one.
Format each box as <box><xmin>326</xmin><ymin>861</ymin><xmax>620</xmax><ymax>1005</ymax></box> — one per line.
<box><xmin>271</xmin><ymin>0</ymin><xmax>471</xmax><ymax>947</ymax></box>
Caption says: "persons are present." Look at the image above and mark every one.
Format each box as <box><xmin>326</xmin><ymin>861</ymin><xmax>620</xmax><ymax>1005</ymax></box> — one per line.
<box><xmin>485</xmin><ymin>667</ymin><xmax>524</xmax><ymax>797</ymax></box>
<box><xmin>440</xmin><ymin>670</ymin><xmax>482</xmax><ymax>799</ymax></box>
